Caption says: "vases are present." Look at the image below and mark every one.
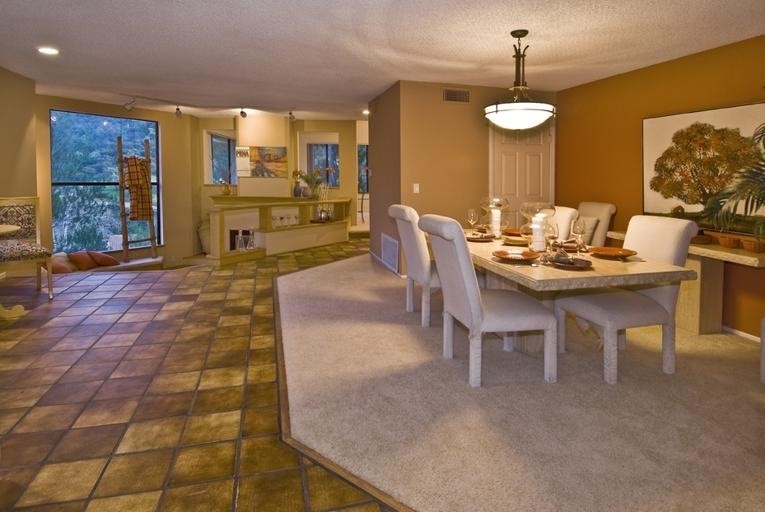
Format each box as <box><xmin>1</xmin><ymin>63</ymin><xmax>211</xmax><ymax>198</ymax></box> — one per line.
<box><xmin>223</xmin><ymin>184</ymin><xmax>230</xmax><ymax>195</ymax></box>
<box><xmin>303</xmin><ymin>184</ymin><xmax>319</xmax><ymax>198</ymax></box>
<box><xmin>294</xmin><ymin>181</ymin><xmax>302</xmax><ymax>197</ymax></box>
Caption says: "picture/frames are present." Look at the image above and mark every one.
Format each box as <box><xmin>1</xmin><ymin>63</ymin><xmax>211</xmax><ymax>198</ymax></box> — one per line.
<box><xmin>642</xmin><ymin>101</ymin><xmax>765</xmax><ymax>224</ymax></box>
<box><xmin>381</xmin><ymin>233</ymin><xmax>398</xmax><ymax>271</ymax></box>
<box><xmin>235</xmin><ymin>146</ymin><xmax>288</xmax><ymax>178</ymax></box>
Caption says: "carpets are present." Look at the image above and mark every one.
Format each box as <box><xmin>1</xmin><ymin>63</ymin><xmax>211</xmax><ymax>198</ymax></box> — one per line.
<box><xmin>277</xmin><ymin>253</ymin><xmax>765</xmax><ymax>512</ymax></box>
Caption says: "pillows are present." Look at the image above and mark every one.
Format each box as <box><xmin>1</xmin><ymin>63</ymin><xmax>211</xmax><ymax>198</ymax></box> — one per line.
<box><xmin>51</xmin><ymin>252</ymin><xmax>79</xmax><ymax>270</ymax></box>
<box><xmin>52</xmin><ymin>255</ymin><xmax>69</xmax><ymax>273</ymax></box>
<box><xmin>87</xmin><ymin>251</ymin><xmax>120</xmax><ymax>266</ymax></box>
<box><xmin>67</xmin><ymin>251</ymin><xmax>99</xmax><ymax>271</ymax></box>
<box><xmin>573</xmin><ymin>216</ymin><xmax>599</xmax><ymax>245</ymax></box>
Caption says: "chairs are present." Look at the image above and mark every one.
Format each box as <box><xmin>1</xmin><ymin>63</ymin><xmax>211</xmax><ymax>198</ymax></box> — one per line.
<box><xmin>540</xmin><ymin>206</ymin><xmax>579</xmax><ymax>240</ymax></box>
<box><xmin>388</xmin><ymin>204</ymin><xmax>486</xmax><ymax>327</ymax></box>
<box><xmin>554</xmin><ymin>215</ymin><xmax>699</xmax><ymax>385</ymax></box>
<box><xmin>0</xmin><ymin>197</ymin><xmax>53</xmax><ymax>299</ymax></box>
<box><xmin>418</xmin><ymin>213</ymin><xmax>557</xmax><ymax>388</ymax></box>
<box><xmin>578</xmin><ymin>202</ymin><xmax>616</xmax><ymax>246</ymax></box>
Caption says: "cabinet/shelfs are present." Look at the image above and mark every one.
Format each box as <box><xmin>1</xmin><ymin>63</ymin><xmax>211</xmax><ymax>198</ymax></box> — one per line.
<box><xmin>206</xmin><ymin>196</ymin><xmax>352</xmax><ymax>266</ymax></box>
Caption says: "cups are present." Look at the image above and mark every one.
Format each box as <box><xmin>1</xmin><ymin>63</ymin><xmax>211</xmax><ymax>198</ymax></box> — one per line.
<box><xmin>544</xmin><ymin>223</ymin><xmax>559</xmax><ymax>256</ymax></box>
<box><xmin>466</xmin><ymin>208</ymin><xmax>479</xmax><ymax>236</ymax></box>
<box><xmin>570</xmin><ymin>219</ymin><xmax>587</xmax><ymax>258</ymax></box>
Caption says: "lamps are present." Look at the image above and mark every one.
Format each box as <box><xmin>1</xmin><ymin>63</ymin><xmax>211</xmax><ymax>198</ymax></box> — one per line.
<box><xmin>175</xmin><ymin>104</ymin><xmax>183</xmax><ymax>120</ymax></box>
<box><xmin>288</xmin><ymin>112</ymin><xmax>295</xmax><ymax>121</ymax></box>
<box><xmin>484</xmin><ymin>29</ymin><xmax>557</xmax><ymax>130</ymax></box>
<box><xmin>123</xmin><ymin>96</ymin><xmax>137</xmax><ymax>112</ymax></box>
<box><xmin>240</xmin><ymin>108</ymin><xmax>247</xmax><ymax>119</ymax></box>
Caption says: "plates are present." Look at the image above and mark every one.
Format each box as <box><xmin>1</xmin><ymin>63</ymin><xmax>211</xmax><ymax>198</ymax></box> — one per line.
<box><xmin>547</xmin><ymin>255</ymin><xmax>593</xmax><ymax>270</ymax></box>
<box><xmin>502</xmin><ymin>239</ymin><xmax>528</xmax><ymax>247</ymax></box>
<box><xmin>588</xmin><ymin>247</ymin><xmax>638</xmax><ymax>259</ymax></box>
<box><xmin>465</xmin><ymin>237</ymin><xmax>494</xmax><ymax>242</ymax></box>
<box><xmin>553</xmin><ymin>245</ymin><xmax>579</xmax><ymax>253</ymax></box>
<box><xmin>501</xmin><ymin>227</ymin><xmax>533</xmax><ymax>236</ymax></box>
<box><xmin>492</xmin><ymin>248</ymin><xmax>542</xmax><ymax>262</ymax></box>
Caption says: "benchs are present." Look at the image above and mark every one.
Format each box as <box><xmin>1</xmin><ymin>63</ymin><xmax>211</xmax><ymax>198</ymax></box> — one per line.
<box><xmin>88</xmin><ymin>256</ymin><xmax>164</xmax><ymax>271</ymax></box>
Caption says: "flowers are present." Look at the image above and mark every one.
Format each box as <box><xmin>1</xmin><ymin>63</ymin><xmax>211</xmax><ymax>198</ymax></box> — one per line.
<box><xmin>292</xmin><ymin>169</ymin><xmax>304</xmax><ymax>181</ymax></box>
<box><xmin>299</xmin><ymin>167</ymin><xmax>335</xmax><ymax>198</ymax></box>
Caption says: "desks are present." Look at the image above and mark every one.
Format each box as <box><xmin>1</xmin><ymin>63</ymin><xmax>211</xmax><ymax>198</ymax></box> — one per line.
<box><xmin>0</xmin><ymin>225</ymin><xmax>24</xmax><ymax>321</ymax></box>
